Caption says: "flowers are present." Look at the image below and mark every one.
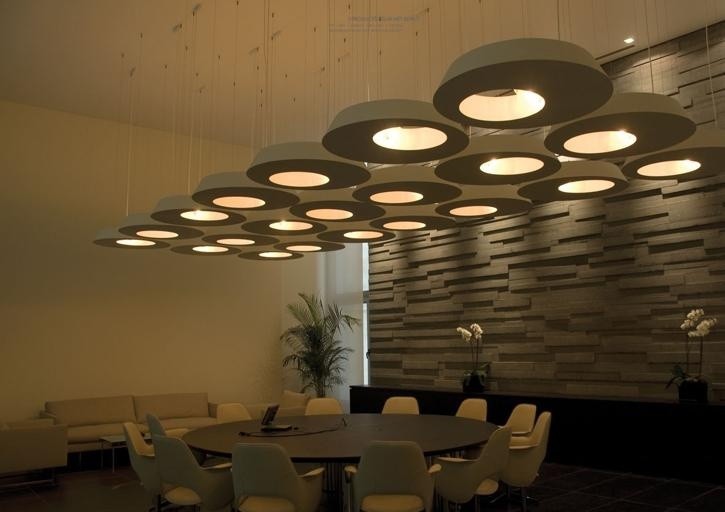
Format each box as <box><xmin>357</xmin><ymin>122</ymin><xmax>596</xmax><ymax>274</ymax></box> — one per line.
<box><xmin>657</xmin><ymin>305</ymin><xmax>718</xmax><ymax>380</ymax></box>
<box><xmin>454</xmin><ymin>320</ymin><xmax>490</xmax><ymax>374</ymax></box>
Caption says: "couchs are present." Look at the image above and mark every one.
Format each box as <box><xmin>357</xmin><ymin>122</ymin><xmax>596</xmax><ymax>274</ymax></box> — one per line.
<box><xmin>249</xmin><ymin>391</ymin><xmax>311</xmax><ymax>417</ymax></box>
<box><xmin>0</xmin><ymin>409</ymin><xmax>68</xmax><ymax>484</ymax></box>
<box><xmin>41</xmin><ymin>392</ymin><xmax>217</xmax><ymax>469</ymax></box>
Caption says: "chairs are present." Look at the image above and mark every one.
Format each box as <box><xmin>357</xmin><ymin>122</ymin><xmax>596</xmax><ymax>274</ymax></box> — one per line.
<box><xmin>496</xmin><ymin>401</ymin><xmax>536</xmax><ymax>437</ymax></box>
<box><xmin>147</xmin><ymin>397</ymin><xmax>204</xmax><ymax>465</ymax></box>
<box><xmin>230</xmin><ymin>443</ymin><xmax>325</xmax><ymax>512</ymax></box>
<box><xmin>122</xmin><ymin>419</ymin><xmax>156</xmax><ymax>488</ymax></box>
<box><xmin>500</xmin><ymin>411</ymin><xmax>552</xmax><ymax>507</ymax></box>
<box><xmin>380</xmin><ymin>395</ymin><xmax>421</xmax><ymax>416</ymax></box>
<box><xmin>432</xmin><ymin>427</ymin><xmax>512</xmax><ymax>503</ymax></box>
<box><xmin>455</xmin><ymin>397</ymin><xmax>488</xmax><ymax>422</ymax></box>
<box><xmin>304</xmin><ymin>395</ymin><xmax>339</xmax><ymax>413</ymax></box>
<box><xmin>212</xmin><ymin>400</ymin><xmax>255</xmax><ymax>421</ymax></box>
<box><xmin>343</xmin><ymin>441</ymin><xmax>441</xmax><ymax>512</ymax></box>
<box><xmin>155</xmin><ymin>437</ymin><xmax>233</xmax><ymax>512</ymax></box>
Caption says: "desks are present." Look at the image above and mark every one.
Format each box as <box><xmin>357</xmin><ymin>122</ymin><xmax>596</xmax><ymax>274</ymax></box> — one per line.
<box><xmin>182</xmin><ymin>413</ymin><xmax>502</xmax><ymax>463</ymax></box>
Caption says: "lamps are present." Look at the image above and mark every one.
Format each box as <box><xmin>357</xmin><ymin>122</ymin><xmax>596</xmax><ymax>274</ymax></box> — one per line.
<box><xmin>433</xmin><ymin>0</ymin><xmax>614</xmax><ymax>131</ymax></box>
<box><xmin>191</xmin><ymin>0</ymin><xmax>301</xmax><ymax>214</ymax></box>
<box><xmin>621</xmin><ymin>1</ymin><xmax>724</xmax><ymax>182</ymax></box>
<box><xmin>288</xmin><ymin>195</ymin><xmax>387</xmax><ymax>223</ymax></box>
<box><xmin>352</xmin><ymin>168</ymin><xmax>464</xmax><ymax>211</ymax></box>
<box><xmin>150</xmin><ymin>197</ymin><xmax>250</xmax><ymax>229</ymax></box>
<box><xmin>517</xmin><ymin>163</ymin><xmax>617</xmax><ymax>202</ymax></box>
<box><xmin>435</xmin><ymin>190</ymin><xmax>533</xmax><ymax>218</ymax></box>
<box><xmin>118</xmin><ymin>32</ymin><xmax>206</xmax><ymax>242</ymax></box>
<box><xmin>93</xmin><ymin>67</ymin><xmax>172</xmax><ymax>251</ymax></box>
<box><xmin>246</xmin><ymin>0</ymin><xmax>371</xmax><ymax>194</ymax></box>
<box><xmin>433</xmin><ymin>134</ymin><xmax>561</xmax><ymax>188</ymax></box>
<box><xmin>368</xmin><ymin>214</ymin><xmax>458</xmax><ymax>232</ymax></box>
<box><xmin>320</xmin><ymin>0</ymin><xmax>470</xmax><ymax>166</ymax></box>
<box><xmin>543</xmin><ymin>1</ymin><xmax>698</xmax><ymax>164</ymax></box>
<box><xmin>316</xmin><ymin>225</ymin><xmax>397</xmax><ymax>244</ymax></box>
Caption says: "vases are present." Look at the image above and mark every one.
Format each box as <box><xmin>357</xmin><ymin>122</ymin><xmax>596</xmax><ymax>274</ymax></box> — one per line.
<box><xmin>662</xmin><ymin>370</ymin><xmax>717</xmax><ymax>406</ymax></box>
<box><xmin>457</xmin><ymin>365</ymin><xmax>487</xmax><ymax>393</ymax></box>
